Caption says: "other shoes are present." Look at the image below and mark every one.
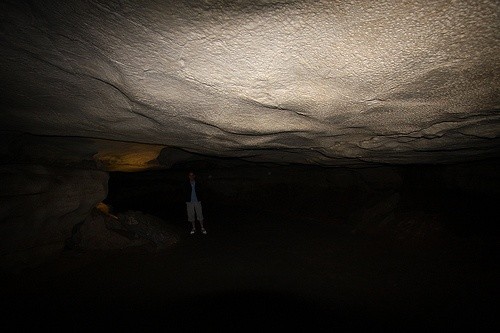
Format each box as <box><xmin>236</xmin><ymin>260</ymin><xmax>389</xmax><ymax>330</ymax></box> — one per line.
<box><xmin>190</xmin><ymin>227</ymin><xmax>196</xmax><ymax>234</ymax></box>
<box><xmin>201</xmin><ymin>228</ymin><xmax>207</xmax><ymax>234</ymax></box>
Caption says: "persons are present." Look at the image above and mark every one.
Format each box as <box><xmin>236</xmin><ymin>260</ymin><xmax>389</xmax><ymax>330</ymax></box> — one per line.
<box><xmin>184</xmin><ymin>171</ymin><xmax>208</xmax><ymax>236</ymax></box>
